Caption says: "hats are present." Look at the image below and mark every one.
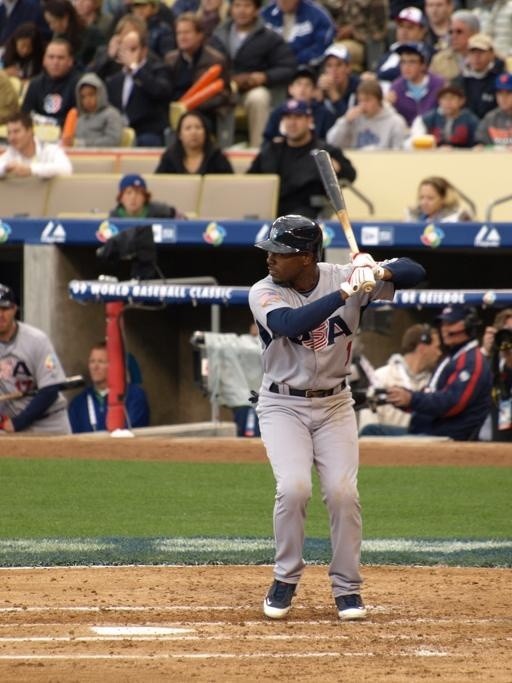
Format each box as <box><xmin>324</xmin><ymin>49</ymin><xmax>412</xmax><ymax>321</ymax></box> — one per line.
<box><xmin>434</xmin><ymin>303</ymin><xmax>475</xmax><ymax>321</ymax></box>
<box><xmin>321</xmin><ymin>44</ymin><xmax>349</xmax><ymax>64</ymax></box>
<box><xmin>120</xmin><ymin>174</ymin><xmax>145</xmax><ymax>192</ymax></box>
<box><xmin>276</xmin><ymin>98</ymin><xmax>312</xmax><ymax>116</ymax></box>
<box><xmin>468</xmin><ymin>35</ymin><xmax>512</xmax><ymax>90</ymax></box>
<box><xmin>388</xmin><ymin>6</ymin><xmax>432</xmax><ymax>62</ymax></box>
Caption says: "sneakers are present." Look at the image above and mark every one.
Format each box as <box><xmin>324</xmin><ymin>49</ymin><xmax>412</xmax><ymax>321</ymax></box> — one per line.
<box><xmin>335</xmin><ymin>594</ymin><xmax>368</xmax><ymax>619</ymax></box>
<box><xmin>264</xmin><ymin>579</ymin><xmax>296</xmax><ymax>619</ymax></box>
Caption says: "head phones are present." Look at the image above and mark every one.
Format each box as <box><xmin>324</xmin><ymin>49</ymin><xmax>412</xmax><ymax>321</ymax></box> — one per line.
<box><xmin>464</xmin><ymin>303</ymin><xmax>484</xmax><ymax>338</ymax></box>
<box><xmin>419</xmin><ymin>323</ymin><xmax>432</xmax><ymax>344</ymax></box>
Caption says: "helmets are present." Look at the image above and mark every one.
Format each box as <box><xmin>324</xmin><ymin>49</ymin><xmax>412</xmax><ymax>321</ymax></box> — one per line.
<box><xmin>254</xmin><ymin>215</ymin><xmax>323</xmax><ymax>262</ymax></box>
<box><xmin>0</xmin><ymin>284</ymin><xmax>16</xmax><ymax>308</ymax></box>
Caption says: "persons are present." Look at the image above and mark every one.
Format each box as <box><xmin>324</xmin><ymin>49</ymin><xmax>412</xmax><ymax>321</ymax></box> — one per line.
<box><xmin>68</xmin><ymin>343</ymin><xmax>149</xmax><ymax>433</ymax></box>
<box><xmin>0</xmin><ymin>285</ymin><xmax>73</xmax><ymax>434</ymax></box>
<box><xmin>1</xmin><ymin>1</ymin><xmax>512</xmax><ymax>220</ymax></box>
<box><xmin>246</xmin><ymin>213</ymin><xmax>429</xmax><ymax>620</ymax></box>
<box><xmin>360</xmin><ymin>304</ymin><xmax>512</xmax><ymax>435</ymax></box>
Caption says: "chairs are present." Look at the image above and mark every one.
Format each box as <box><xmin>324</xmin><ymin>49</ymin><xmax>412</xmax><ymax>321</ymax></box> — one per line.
<box><xmin>0</xmin><ymin>146</ymin><xmax>283</xmax><ymax>223</ymax></box>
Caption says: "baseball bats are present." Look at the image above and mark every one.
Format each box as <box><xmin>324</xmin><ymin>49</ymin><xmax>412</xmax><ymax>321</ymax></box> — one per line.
<box><xmin>316</xmin><ymin>150</ymin><xmax>376</xmax><ymax>293</ymax></box>
<box><xmin>1</xmin><ymin>376</ymin><xmax>85</xmax><ymax>401</ymax></box>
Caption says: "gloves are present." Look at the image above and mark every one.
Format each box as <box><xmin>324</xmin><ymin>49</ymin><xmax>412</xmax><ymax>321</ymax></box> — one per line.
<box><xmin>340</xmin><ymin>251</ymin><xmax>384</xmax><ymax>296</ymax></box>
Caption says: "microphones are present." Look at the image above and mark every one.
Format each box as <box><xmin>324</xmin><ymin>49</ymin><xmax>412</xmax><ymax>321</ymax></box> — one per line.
<box><xmin>448</xmin><ymin>329</ymin><xmax>467</xmax><ymax>336</ymax></box>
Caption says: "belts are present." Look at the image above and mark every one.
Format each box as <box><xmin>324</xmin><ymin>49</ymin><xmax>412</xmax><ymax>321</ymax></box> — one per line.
<box><xmin>270</xmin><ymin>380</ymin><xmax>346</xmax><ymax>397</ymax></box>
